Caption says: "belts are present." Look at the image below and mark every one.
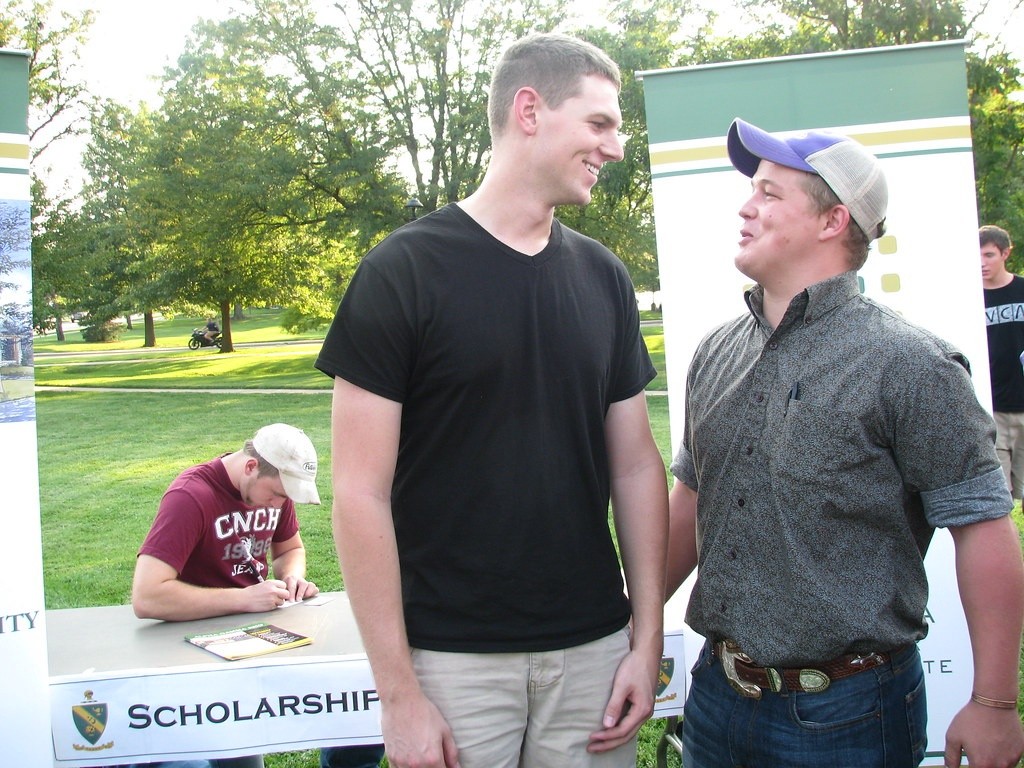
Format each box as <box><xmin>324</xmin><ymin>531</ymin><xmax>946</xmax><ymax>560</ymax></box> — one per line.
<box><xmin>711</xmin><ymin>641</ymin><xmax>909</xmax><ymax>700</ymax></box>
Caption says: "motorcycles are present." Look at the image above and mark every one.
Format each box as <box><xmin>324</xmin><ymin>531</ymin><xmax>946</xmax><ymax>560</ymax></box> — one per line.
<box><xmin>188</xmin><ymin>328</ymin><xmax>223</xmax><ymax>350</ymax></box>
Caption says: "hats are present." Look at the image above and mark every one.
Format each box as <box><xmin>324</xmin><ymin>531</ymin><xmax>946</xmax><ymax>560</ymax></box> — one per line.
<box><xmin>253</xmin><ymin>423</ymin><xmax>322</xmax><ymax>505</ymax></box>
<box><xmin>727</xmin><ymin>117</ymin><xmax>888</xmax><ymax>245</ymax></box>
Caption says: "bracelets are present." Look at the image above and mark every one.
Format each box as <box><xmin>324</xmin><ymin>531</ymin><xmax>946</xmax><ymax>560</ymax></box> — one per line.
<box><xmin>970</xmin><ymin>688</ymin><xmax>1018</xmax><ymax>710</ymax></box>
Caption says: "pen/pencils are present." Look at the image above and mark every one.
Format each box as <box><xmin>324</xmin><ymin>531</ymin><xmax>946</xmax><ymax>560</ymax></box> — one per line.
<box><xmin>247</xmin><ymin>561</ymin><xmax>264</xmax><ymax>583</ymax></box>
<box><xmin>780</xmin><ymin>380</ymin><xmax>798</xmax><ymax>419</ymax></box>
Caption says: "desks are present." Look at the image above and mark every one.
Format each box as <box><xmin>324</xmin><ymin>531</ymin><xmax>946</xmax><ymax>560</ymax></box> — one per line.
<box><xmin>46</xmin><ymin>570</ymin><xmax>685</xmax><ymax>768</ymax></box>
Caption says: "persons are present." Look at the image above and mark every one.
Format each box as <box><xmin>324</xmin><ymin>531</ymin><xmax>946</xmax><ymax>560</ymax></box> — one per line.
<box><xmin>978</xmin><ymin>225</ymin><xmax>1023</xmax><ymax>505</ymax></box>
<box><xmin>315</xmin><ymin>31</ymin><xmax>673</xmax><ymax>767</ymax></box>
<box><xmin>134</xmin><ymin>421</ymin><xmax>387</xmax><ymax>767</ymax></box>
<box><xmin>627</xmin><ymin>114</ymin><xmax>1023</xmax><ymax>768</ymax></box>
<box><xmin>201</xmin><ymin>317</ymin><xmax>220</xmax><ymax>343</ymax></box>
